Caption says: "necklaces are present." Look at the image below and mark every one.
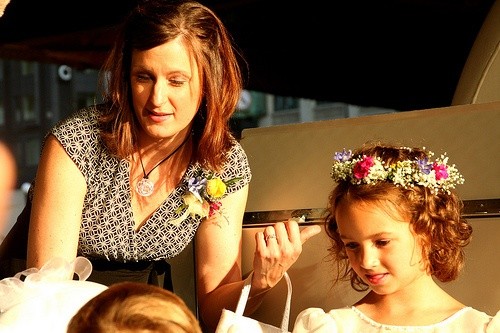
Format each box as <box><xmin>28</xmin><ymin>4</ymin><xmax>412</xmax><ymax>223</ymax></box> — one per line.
<box><xmin>135</xmin><ymin>140</ymin><xmax>189</xmax><ymax>197</ymax></box>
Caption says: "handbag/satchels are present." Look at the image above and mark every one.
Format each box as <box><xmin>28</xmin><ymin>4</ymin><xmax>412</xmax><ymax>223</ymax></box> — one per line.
<box><xmin>214</xmin><ymin>269</ymin><xmax>293</xmax><ymax>333</ymax></box>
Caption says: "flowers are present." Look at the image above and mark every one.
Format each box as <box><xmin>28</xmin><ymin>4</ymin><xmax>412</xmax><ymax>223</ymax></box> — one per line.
<box><xmin>330</xmin><ymin>148</ymin><xmax>465</xmax><ymax>194</ymax></box>
<box><xmin>169</xmin><ymin>178</ymin><xmax>241</xmax><ymax>228</ymax></box>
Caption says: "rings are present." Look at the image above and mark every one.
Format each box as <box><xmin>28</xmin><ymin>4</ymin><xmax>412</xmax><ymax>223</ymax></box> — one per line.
<box><xmin>266</xmin><ymin>235</ymin><xmax>276</xmax><ymax>240</ymax></box>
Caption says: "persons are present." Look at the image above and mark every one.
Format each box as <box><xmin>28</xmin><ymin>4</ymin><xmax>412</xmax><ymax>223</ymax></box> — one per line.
<box><xmin>291</xmin><ymin>143</ymin><xmax>500</xmax><ymax>333</ymax></box>
<box><xmin>0</xmin><ymin>0</ymin><xmax>322</xmax><ymax>333</ymax></box>
